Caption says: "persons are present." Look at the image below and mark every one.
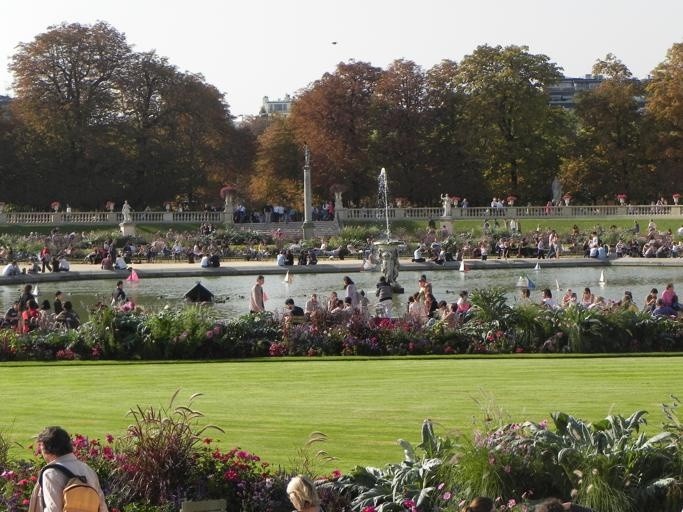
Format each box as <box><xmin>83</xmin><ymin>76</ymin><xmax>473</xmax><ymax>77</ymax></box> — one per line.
<box><xmin>460</xmin><ymin>495</ymin><xmax>496</xmax><ymax>512</ymax></box>
<box><xmin>283</xmin><ymin>274</ymin><xmax>683</xmax><ymax>327</ymax></box>
<box><xmin>27</xmin><ymin>425</ymin><xmax>109</xmax><ymax>511</ymax></box>
<box><xmin>0</xmin><ymin>281</ymin><xmax>127</xmax><ymax>336</ymax></box>
<box><xmin>249</xmin><ymin>275</ymin><xmax>265</xmax><ymax>313</ymax></box>
<box><xmin>537</xmin><ymin>498</ymin><xmax>592</xmax><ymax>512</ymax></box>
<box><xmin>286</xmin><ymin>472</ymin><xmax>323</xmax><ymax>511</ymax></box>
<box><xmin>0</xmin><ymin>191</ymin><xmax>682</xmax><ymax>277</ymax></box>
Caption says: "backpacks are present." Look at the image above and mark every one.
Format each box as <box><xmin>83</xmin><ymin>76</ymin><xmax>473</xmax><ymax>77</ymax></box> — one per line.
<box><xmin>39</xmin><ymin>462</ymin><xmax>103</xmax><ymax>512</ymax></box>
